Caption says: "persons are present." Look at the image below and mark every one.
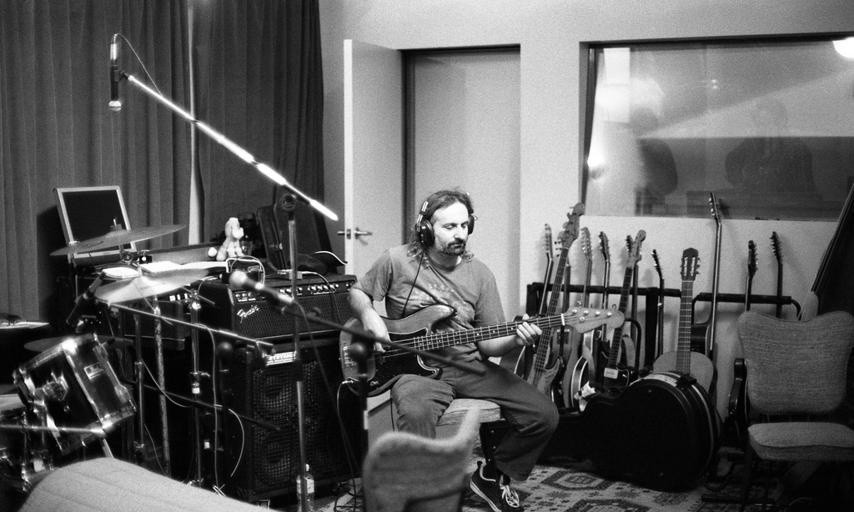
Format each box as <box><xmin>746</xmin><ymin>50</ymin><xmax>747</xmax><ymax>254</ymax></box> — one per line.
<box><xmin>345</xmin><ymin>189</ymin><xmax>560</xmax><ymax>511</ymax></box>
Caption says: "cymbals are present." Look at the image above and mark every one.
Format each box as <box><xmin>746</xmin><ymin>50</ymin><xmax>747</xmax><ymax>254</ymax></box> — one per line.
<box><xmin>0</xmin><ymin>321</ymin><xmax>49</xmax><ymax>330</ymax></box>
<box><xmin>49</xmin><ymin>225</ymin><xmax>186</xmax><ymax>260</ymax></box>
<box><xmin>23</xmin><ymin>334</ymin><xmax>133</xmax><ymax>353</ymax></box>
<box><xmin>95</xmin><ymin>269</ymin><xmax>209</xmax><ymax>303</ymax></box>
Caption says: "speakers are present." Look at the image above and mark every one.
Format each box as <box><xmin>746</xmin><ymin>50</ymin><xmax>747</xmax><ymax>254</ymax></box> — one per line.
<box><xmin>192</xmin><ymin>280</ymin><xmax>369</xmax><ymax>505</ymax></box>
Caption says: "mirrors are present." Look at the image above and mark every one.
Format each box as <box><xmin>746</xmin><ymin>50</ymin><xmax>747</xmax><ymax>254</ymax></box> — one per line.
<box><xmin>576</xmin><ymin>30</ymin><xmax>851</xmax><ymax>225</ymax></box>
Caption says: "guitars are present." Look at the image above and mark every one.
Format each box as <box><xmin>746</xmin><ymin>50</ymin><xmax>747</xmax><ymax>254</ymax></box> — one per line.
<box><xmin>499</xmin><ymin>224</ymin><xmax>555</xmax><ymax>382</ymax></box>
<box><xmin>517</xmin><ymin>200</ymin><xmax>586</xmax><ymax>401</ymax></box>
<box><xmin>546</xmin><ymin>227</ymin><xmax>666</xmax><ymax>398</ymax></box>
<box><xmin>653</xmin><ymin>247</ymin><xmax>718</xmax><ymax>401</ymax></box>
<box><xmin>725</xmin><ymin>241</ymin><xmax>773</xmax><ymax>427</ymax></box>
<box><xmin>688</xmin><ymin>192</ymin><xmax>730</xmax><ymax>399</ymax></box>
<box><xmin>339</xmin><ymin>301</ymin><xmax>625</xmax><ymax>398</ymax></box>
<box><xmin>765</xmin><ymin>232</ymin><xmax>813</xmax><ymax>425</ymax></box>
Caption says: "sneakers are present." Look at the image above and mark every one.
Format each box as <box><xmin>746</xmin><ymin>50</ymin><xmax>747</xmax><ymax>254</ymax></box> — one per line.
<box><xmin>469</xmin><ymin>461</ymin><xmax>524</xmax><ymax>512</ymax></box>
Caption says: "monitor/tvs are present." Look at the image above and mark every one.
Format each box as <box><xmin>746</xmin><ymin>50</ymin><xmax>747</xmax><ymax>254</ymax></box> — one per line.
<box><xmin>55</xmin><ymin>184</ymin><xmax>139</xmax><ymax>259</ymax></box>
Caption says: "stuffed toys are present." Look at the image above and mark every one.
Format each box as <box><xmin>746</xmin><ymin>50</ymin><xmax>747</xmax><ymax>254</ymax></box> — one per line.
<box><xmin>208</xmin><ymin>217</ymin><xmax>245</xmax><ymax>261</ymax></box>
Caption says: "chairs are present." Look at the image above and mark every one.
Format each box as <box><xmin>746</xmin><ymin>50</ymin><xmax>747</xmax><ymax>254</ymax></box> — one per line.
<box><xmin>358</xmin><ymin>406</ymin><xmax>481</xmax><ymax>512</ymax></box>
<box><xmin>737</xmin><ymin>306</ymin><xmax>854</xmax><ymax>510</ymax></box>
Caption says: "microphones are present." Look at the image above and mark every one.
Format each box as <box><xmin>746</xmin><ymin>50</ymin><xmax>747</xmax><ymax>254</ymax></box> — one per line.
<box><xmin>229</xmin><ymin>269</ymin><xmax>299</xmax><ymax>308</ymax></box>
<box><xmin>107</xmin><ymin>40</ymin><xmax>125</xmax><ymax>115</ymax></box>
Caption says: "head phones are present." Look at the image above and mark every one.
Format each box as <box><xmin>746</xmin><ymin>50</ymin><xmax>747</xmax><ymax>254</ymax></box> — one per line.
<box><xmin>415</xmin><ymin>192</ymin><xmax>476</xmax><ymax>246</ymax></box>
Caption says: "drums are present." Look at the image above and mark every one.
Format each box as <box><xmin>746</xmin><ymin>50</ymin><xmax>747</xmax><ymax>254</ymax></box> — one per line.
<box><xmin>0</xmin><ymin>393</ymin><xmax>43</xmax><ymax>493</ymax></box>
<box><xmin>11</xmin><ymin>331</ymin><xmax>138</xmax><ymax>465</ymax></box>
